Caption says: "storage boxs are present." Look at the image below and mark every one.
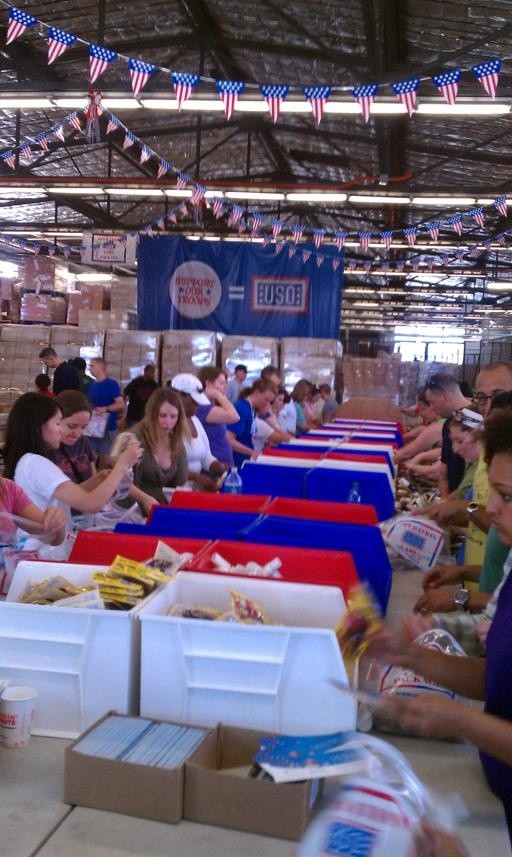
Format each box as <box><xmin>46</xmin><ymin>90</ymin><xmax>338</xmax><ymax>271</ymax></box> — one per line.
<box><xmin>0</xmin><ymin>252</ymin><xmax>412</xmax><ymax>838</ymax></box>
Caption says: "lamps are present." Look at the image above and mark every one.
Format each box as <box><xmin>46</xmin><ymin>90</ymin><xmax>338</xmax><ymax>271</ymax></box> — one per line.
<box><xmin>1</xmin><ymin>90</ymin><xmax>511</xmax><ymax>117</ymax></box>
<box><xmin>1</xmin><ymin>185</ymin><xmax>512</xmax><ymax>208</ymax></box>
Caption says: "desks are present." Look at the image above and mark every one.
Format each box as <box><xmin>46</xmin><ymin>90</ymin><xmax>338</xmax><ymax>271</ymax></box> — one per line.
<box><xmin>0</xmin><ymin>566</ymin><xmax>512</xmax><ymax>856</ymax></box>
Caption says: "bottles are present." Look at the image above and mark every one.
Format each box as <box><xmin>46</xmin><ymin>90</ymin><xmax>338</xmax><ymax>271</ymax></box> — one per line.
<box><xmin>224</xmin><ymin>467</ymin><xmax>242</xmax><ymax>492</ymax></box>
<box><xmin>347</xmin><ymin>481</ymin><xmax>362</xmax><ymax>503</ymax></box>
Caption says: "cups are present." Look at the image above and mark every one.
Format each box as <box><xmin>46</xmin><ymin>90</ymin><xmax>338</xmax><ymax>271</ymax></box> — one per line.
<box><xmin>2</xmin><ymin>686</ymin><xmax>40</xmax><ymax>748</ymax></box>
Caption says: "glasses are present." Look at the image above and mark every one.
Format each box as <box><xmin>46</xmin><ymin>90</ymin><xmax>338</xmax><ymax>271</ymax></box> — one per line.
<box><xmin>472</xmin><ymin>394</ymin><xmax>496</xmax><ymax>405</ymax></box>
<box><xmin>453</xmin><ymin>408</ymin><xmax>480</xmax><ymax>423</ymax></box>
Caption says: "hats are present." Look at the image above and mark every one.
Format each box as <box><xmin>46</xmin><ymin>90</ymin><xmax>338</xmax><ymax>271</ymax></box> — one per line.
<box><xmin>171</xmin><ymin>373</ymin><xmax>211</xmax><ymax>406</ymax></box>
